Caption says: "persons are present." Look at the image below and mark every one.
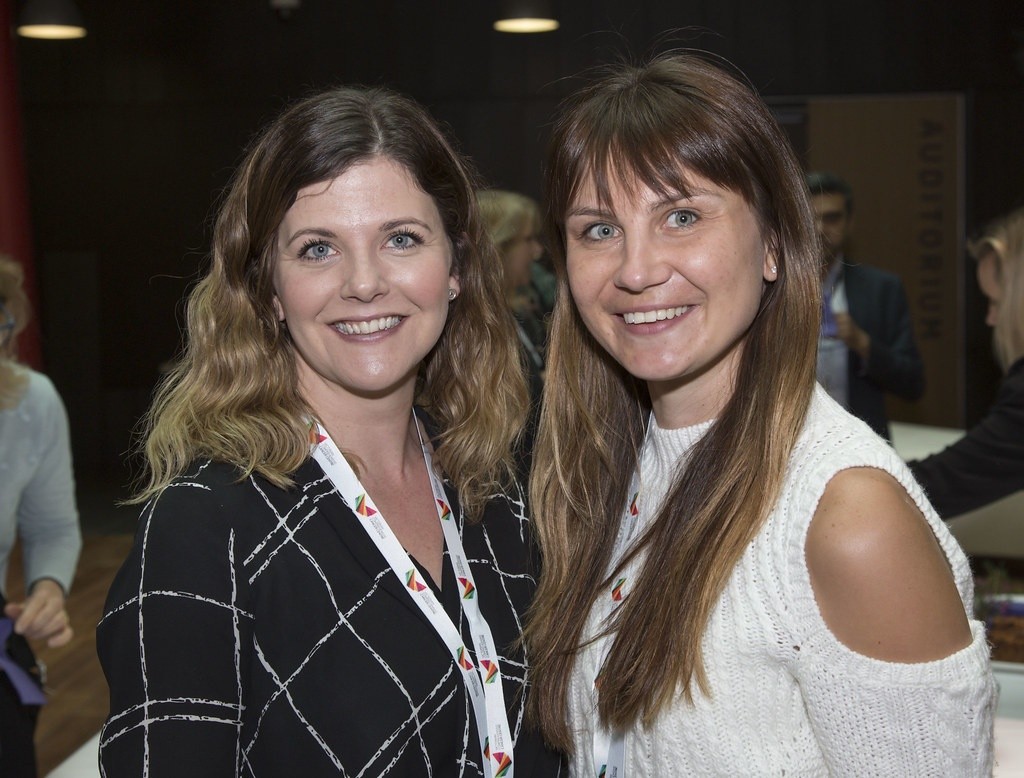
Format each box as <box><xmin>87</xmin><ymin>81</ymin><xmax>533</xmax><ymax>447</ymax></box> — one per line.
<box><xmin>510</xmin><ymin>48</ymin><xmax>993</xmax><ymax>778</ymax></box>
<box><xmin>94</xmin><ymin>88</ymin><xmax>570</xmax><ymax>778</ymax></box>
<box><xmin>475</xmin><ymin>173</ymin><xmax>1024</xmax><ymax>522</ymax></box>
<box><xmin>0</xmin><ymin>260</ymin><xmax>82</xmax><ymax>778</ymax></box>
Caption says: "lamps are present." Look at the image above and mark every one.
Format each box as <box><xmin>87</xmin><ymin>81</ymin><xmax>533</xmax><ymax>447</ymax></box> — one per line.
<box><xmin>493</xmin><ymin>0</ymin><xmax>561</xmax><ymax>33</ymax></box>
<box><xmin>16</xmin><ymin>0</ymin><xmax>91</xmax><ymax>40</ymax></box>
